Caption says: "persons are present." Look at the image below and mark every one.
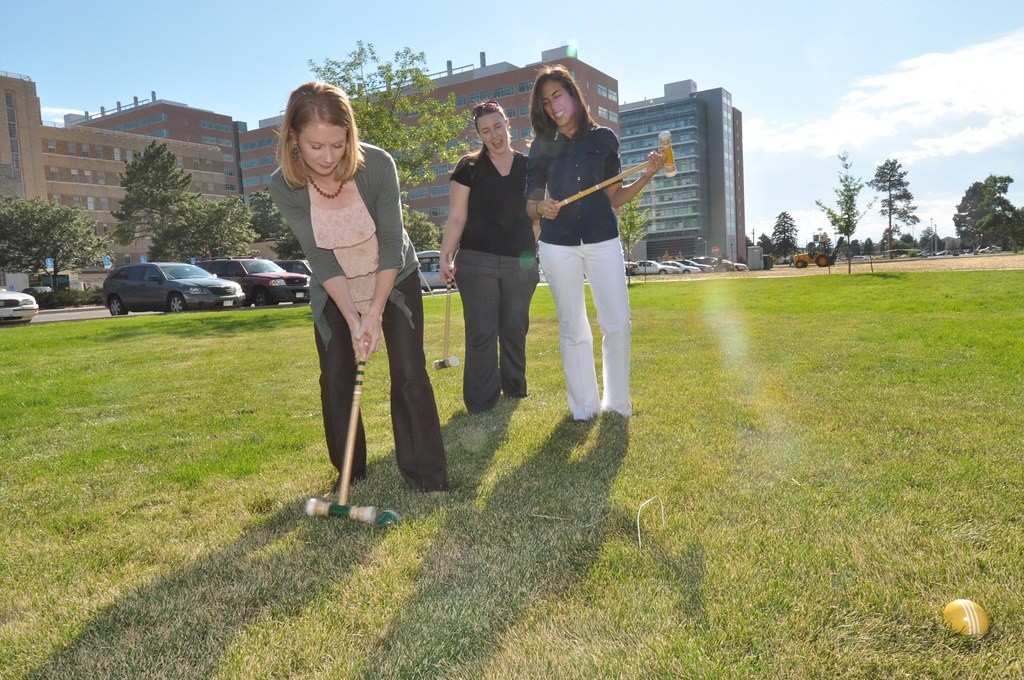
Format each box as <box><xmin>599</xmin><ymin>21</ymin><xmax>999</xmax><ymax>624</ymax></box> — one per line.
<box><xmin>789</xmin><ymin>252</ymin><xmax>794</xmax><ymax>267</ymax></box>
<box><xmin>522</xmin><ymin>66</ymin><xmax>667</xmax><ymax>422</ymax></box>
<box><xmin>264</xmin><ymin>83</ymin><xmax>455</xmax><ymax>498</ymax></box>
<box><xmin>438</xmin><ymin>100</ymin><xmax>539</xmax><ymax>418</ymax></box>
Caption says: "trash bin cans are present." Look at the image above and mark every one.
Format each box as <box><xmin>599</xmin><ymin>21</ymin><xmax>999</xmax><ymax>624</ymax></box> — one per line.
<box><xmin>763</xmin><ymin>255</ymin><xmax>773</xmax><ymax>270</ymax></box>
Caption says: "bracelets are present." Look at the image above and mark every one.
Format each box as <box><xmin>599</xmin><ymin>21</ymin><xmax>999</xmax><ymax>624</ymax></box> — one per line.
<box><xmin>535</xmin><ymin>201</ymin><xmax>543</xmax><ymax>215</ymax></box>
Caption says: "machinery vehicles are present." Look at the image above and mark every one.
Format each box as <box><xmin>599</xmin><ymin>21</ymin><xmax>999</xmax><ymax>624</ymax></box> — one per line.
<box><xmin>791</xmin><ymin>236</ymin><xmax>844</xmax><ymax>269</ymax></box>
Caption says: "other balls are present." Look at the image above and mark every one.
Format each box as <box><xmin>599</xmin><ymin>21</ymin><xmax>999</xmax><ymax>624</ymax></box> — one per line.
<box><xmin>376</xmin><ymin>509</ymin><xmax>400</xmax><ymax>527</ymax></box>
<box><xmin>945</xmin><ymin>599</ymin><xmax>989</xmax><ymax>640</ymax></box>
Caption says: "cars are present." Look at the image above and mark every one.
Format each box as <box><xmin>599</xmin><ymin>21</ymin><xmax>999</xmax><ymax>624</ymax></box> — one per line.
<box><xmin>660</xmin><ymin>261</ymin><xmax>701</xmax><ymax>274</ymax></box>
<box><xmin>102</xmin><ymin>262</ymin><xmax>245</xmax><ymax>317</ymax></box>
<box><xmin>22</xmin><ymin>287</ymin><xmax>54</xmax><ymax>294</ymax></box>
<box><xmin>0</xmin><ymin>287</ymin><xmax>40</xmax><ymax>325</ymax></box>
<box><xmin>635</xmin><ymin>260</ymin><xmax>680</xmax><ymax>275</ymax></box>
<box><xmin>832</xmin><ymin>243</ymin><xmax>1003</xmax><ymax>261</ymax></box>
<box><xmin>676</xmin><ymin>260</ymin><xmax>713</xmax><ymax>273</ymax></box>
<box><xmin>689</xmin><ymin>256</ymin><xmax>748</xmax><ymax>274</ymax></box>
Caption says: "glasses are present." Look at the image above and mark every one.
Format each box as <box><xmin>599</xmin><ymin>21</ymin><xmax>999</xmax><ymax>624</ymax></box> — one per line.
<box><xmin>472</xmin><ymin>101</ymin><xmax>497</xmax><ymax>117</ymax></box>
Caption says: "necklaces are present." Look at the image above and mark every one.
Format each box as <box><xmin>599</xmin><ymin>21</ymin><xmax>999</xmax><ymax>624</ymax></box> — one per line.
<box><xmin>303</xmin><ymin>174</ymin><xmax>344</xmax><ymax>198</ymax></box>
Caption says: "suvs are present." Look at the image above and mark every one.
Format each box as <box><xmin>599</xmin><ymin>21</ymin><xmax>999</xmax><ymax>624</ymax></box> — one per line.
<box><xmin>272</xmin><ymin>258</ymin><xmax>313</xmax><ymax>278</ymax></box>
<box><xmin>624</xmin><ymin>261</ymin><xmax>639</xmax><ymax>276</ymax></box>
<box><xmin>194</xmin><ymin>256</ymin><xmax>312</xmax><ymax>306</ymax></box>
<box><xmin>415</xmin><ymin>250</ymin><xmax>458</xmax><ymax>292</ymax></box>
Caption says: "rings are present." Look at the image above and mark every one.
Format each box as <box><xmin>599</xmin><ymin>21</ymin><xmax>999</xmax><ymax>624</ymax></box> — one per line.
<box><xmin>547</xmin><ymin>208</ymin><xmax>552</xmax><ymax>212</ymax></box>
